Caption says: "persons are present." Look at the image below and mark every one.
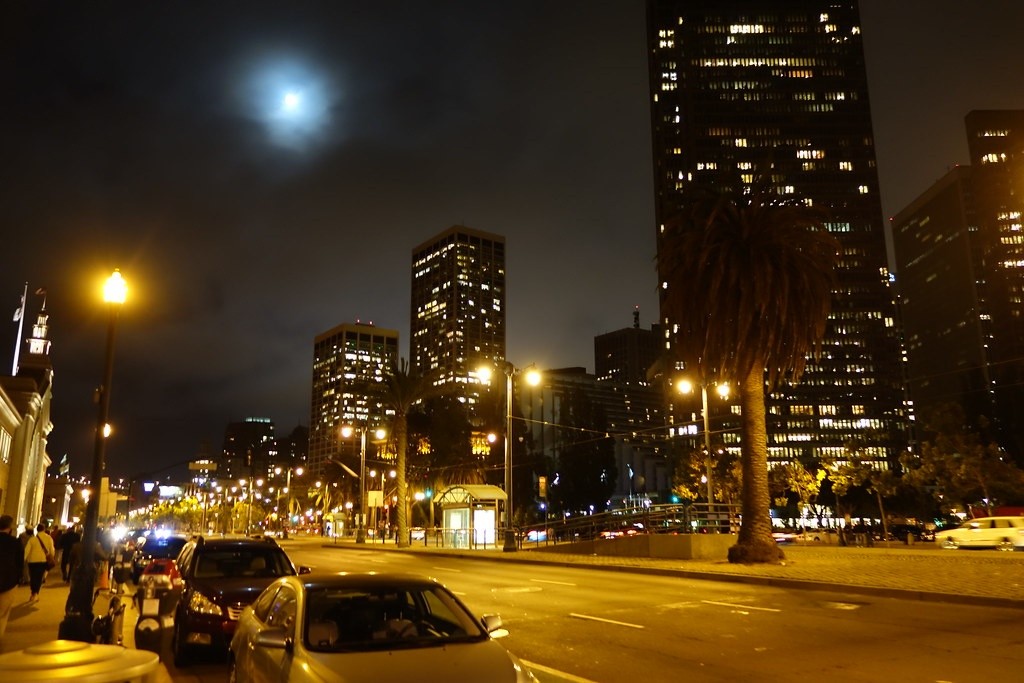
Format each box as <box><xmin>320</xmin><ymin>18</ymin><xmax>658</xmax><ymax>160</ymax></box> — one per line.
<box><xmin>0</xmin><ymin>515</ymin><xmax>24</xmax><ymax>650</ymax></box>
<box><xmin>16</xmin><ymin>512</ymin><xmax>144</xmax><ymax>601</ymax></box>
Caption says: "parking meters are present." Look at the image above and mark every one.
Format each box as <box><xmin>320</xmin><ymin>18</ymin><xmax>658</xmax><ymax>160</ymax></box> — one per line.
<box><xmin>132</xmin><ymin>558</ymin><xmax>184</xmax><ymax>659</ymax></box>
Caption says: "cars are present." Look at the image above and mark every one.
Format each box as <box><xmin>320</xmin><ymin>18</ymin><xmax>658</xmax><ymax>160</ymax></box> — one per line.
<box><xmin>934</xmin><ymin>516</ymin><xmax>1024</xmax><ymax>551</ymax></box>
<box><xmin>797</xmin><ymin>527</ymin><xmax>847</xmax><ymax>545</ymax></box>
<box><xmin>228</xmin><ymin>571</ymin><xmax>541</xmax><ymax>683</ymax></box>
<box><xmin>104</xmin><ymin>522</ymin><xmax>187</xmax><ymax>585</ymax></box>
<box><xmin>888</xmin><ymin>523</ymin><xmax>935</xmax><ymax>543</ymax></box>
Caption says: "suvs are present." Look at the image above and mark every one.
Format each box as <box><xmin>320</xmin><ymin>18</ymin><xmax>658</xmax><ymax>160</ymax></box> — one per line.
<box><xmin>169</xmin><ymin>533</ymin><xmax>312</xmax><ymax>666</ymax></box>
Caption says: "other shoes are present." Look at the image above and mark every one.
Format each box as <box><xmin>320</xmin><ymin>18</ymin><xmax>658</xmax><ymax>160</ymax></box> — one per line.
<box><xmin>18</xmin><ymin>582</ymin><xmax>23</xmax><ymax>587</ymax></box>
<box><xmin>24</xmin><ymin>581</ymin><xmax>29</xmax><ymax>585</ymax></box>
<box><xmin>36</xmin><ymin>597</ymin><xmax>39</xmax><ymax>602</ymax></box>
<box><xmin>30</xmin><ymin>596</ymin><xmax>34</xmax><ymax>602</ymax></box>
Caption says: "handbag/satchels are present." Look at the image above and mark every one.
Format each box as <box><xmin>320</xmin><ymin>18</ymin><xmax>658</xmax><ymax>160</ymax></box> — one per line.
<box><xmin>46</xmin><ymin>554</ymin><xmax>56</xmax><ymax>568</ymax></box>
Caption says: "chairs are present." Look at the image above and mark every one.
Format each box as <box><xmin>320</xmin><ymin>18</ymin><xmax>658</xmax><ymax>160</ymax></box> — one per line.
<box><xmin>197</xmin><ymin>559</ymin><xmax>224</xmax><ymax>577</ymax></box>
<box><xmin>371</xmin><ymin>592</ymin><xmax>418</xmax><ymax>639</ymax></box>
<box><xmin>242</xmin><ymin>557</ymin><xmax>267</xmax><ymax>576</ymax></box>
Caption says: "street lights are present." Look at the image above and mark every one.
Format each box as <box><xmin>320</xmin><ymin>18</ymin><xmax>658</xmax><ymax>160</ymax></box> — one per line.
<box><xmin>240</xmin><ymin>476</ymin><xmax>263</xmax><ymax>537</ymax></box>
<box><xmin>197</xmin><ymin>491</ymin><xmax>215</xmax><ymax>536</ymax></box>
<box><xmin>216</xmin><ymin>484</ymin><xmax>238</xmax><ymax>535</ymax></box>
<box><xmin>678</xmin><ymin>377</ymin><xmax>730</xmax><ymax>532</ymax></box>
<box><xmin>481</xmin><ymin>362</ymin><xmax>539</xmax><ymax>553</ymax></box>
<box><xmin>342</xmin><ymin>426</ymin><xmax>386</xmax><ymax>542</ymax></box>
<box><xmin>58</xmin><ymin>269</ymin><xmax>130</xmax><ymax>643</ymax></box>
<box><xmin>277</xmin><ymin>467</ymin><xmax>303</xmax><ymax>539</ymax></box>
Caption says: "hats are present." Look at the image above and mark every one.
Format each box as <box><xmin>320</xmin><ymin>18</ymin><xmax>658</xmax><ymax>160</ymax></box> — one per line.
<box><xmin>26</xmin><ymin>525</ymin><xmax>32</xmax><ymax>530</ymax></box>
